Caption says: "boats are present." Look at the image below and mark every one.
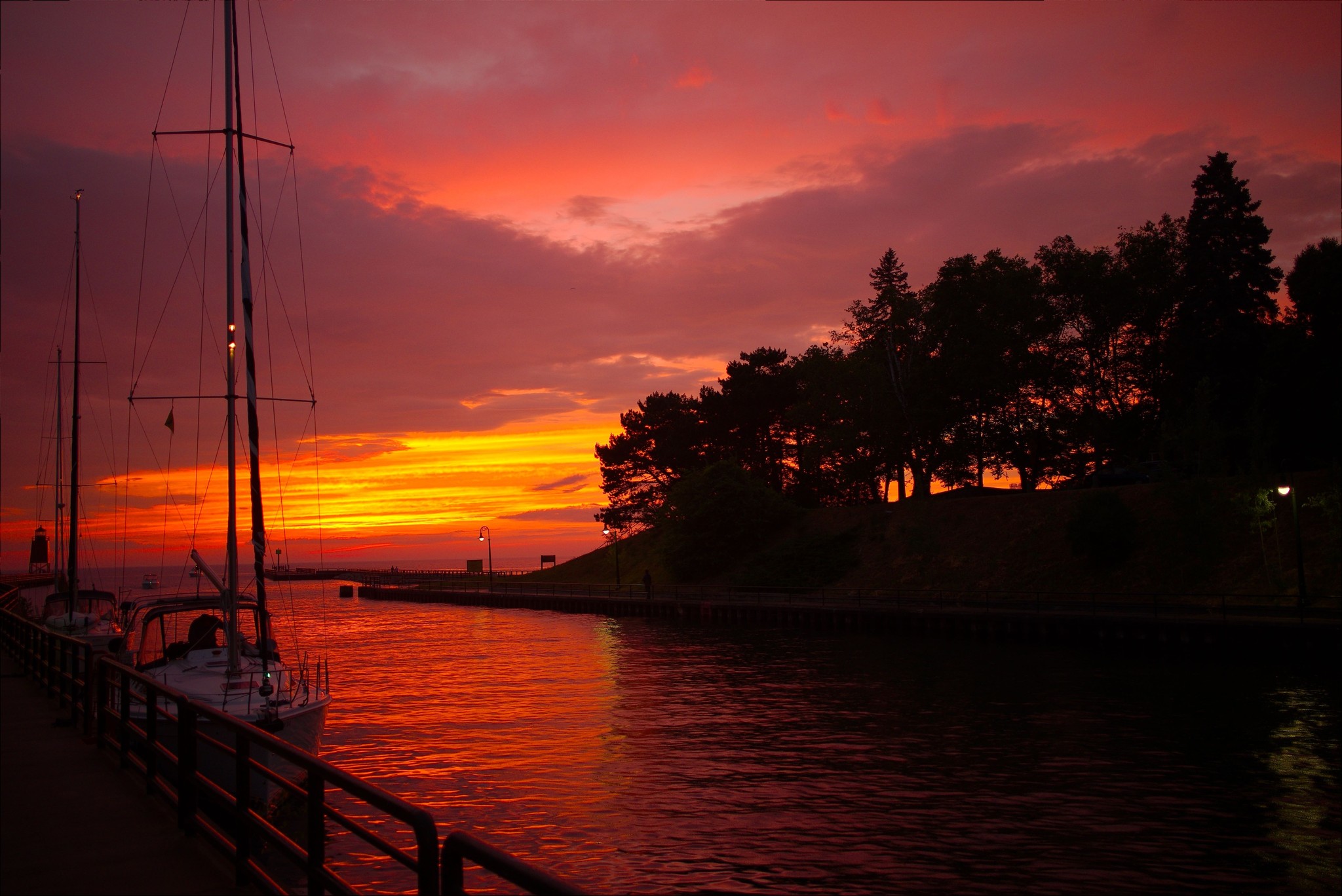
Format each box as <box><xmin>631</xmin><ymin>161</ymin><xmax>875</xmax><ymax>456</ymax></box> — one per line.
<box><xmin>141</xmin><ymin>574</ymin><xmax>159</xmax><ymax>589</ymax></box>
<box><xmin>188</xmin><ymin>565</ymin><xmax>205</xmax><ymax>578</ymax></box>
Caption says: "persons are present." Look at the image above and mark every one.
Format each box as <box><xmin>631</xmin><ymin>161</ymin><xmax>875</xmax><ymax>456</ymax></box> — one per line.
<box><xmin>395</xmin><ymin>566</ymin><xmax>398</xmax><ymax>570</ymax></box>
<box><xmin>272</xmin><ymin>565</ymin><xmax>277</xmax><ymax>571</ymax></box>
<box><xmin>188</xmin><ymin>614</ymin><xmax>223</xmax><ymax>651</ymax></box>
<box><xmin>279</xmin><ymin>565</ymin><xmax>286</xmax><ymax>571</ymax></box>
<box><xmin>391</xmin><ymin>566</ymin><xmax>394</xmax><ymax>575</ymax></box>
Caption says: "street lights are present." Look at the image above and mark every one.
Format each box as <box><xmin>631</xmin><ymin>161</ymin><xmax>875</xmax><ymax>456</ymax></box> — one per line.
<box><xmin>1274</xmin><ymin>464</ymin><xmax>1309</xmax><ymax>608</ymax></box>
<box><xmin>601</xmin><ymin>512</ymin><xmax>622</xmax><ymax>591</ymax></box>
<box><xmin>477</xmin><ymin>525</ymin><xmax>494</xmax><ymax>592</ymax></box>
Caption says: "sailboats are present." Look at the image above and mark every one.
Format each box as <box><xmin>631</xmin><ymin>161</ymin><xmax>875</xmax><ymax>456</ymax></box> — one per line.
<box><xmin>111</xmin><ymin>0</ymin><xmax>335</xmax><ymax>774</ymax></box>
<box><xmin>0</xmin><ymin>184</ymin><xmax>132</xmax><ymax>654</ymax></box>
